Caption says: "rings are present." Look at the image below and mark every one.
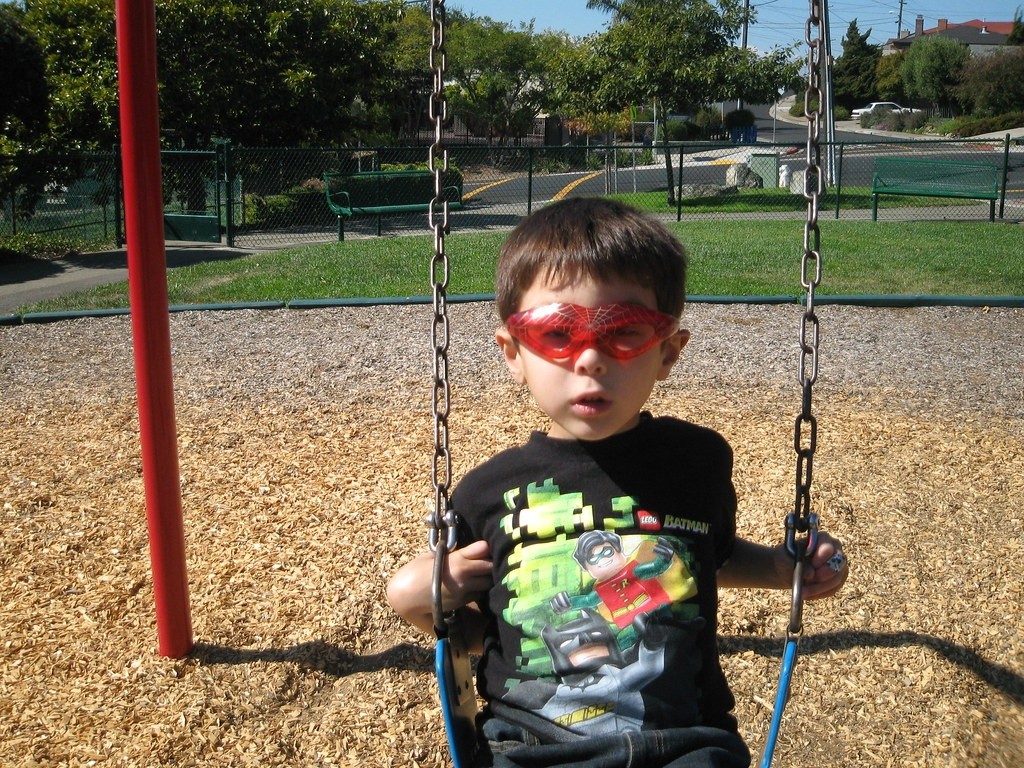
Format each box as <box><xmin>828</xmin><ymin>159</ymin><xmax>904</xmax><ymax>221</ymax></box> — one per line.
<box><xmin>826</xmin><ymin>549</ymin><xmax>847</xmax><ymax>573</ymax></box>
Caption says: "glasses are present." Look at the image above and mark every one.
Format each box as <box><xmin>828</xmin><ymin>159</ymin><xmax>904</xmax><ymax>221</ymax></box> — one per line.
<box><xmin>504</xmin><ymin>302</ymin><xmax>682</xmax><ymax>359</ymax></box>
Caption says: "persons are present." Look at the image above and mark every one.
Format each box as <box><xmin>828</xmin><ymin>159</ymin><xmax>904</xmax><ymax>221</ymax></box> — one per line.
<box><xmin>642</xmin><ymin>127</ymin><xmax>653</xmax><ymax>152</ymax></box>
<box><xmin>387</xmin><ymin>197</ymin><xmax>848</xmax><ymax>768</ymax></box>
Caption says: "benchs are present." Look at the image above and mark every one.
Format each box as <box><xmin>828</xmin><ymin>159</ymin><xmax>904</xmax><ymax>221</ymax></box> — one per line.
<box><xmin>870</xmin><ymin>156</ymin><xmax>999</xmax><ymax>222</ymax></box>
<box><xmin>323</xmin><ymin>169</ymin><xmax>462</xmax><ymax>241</ymax></box>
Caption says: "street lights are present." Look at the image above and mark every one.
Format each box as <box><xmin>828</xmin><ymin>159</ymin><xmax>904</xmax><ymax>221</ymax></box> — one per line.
<box><xmin>888</xmin><ymin>11</ymin><xmax>902</xmax><ymax>40</ymax></box>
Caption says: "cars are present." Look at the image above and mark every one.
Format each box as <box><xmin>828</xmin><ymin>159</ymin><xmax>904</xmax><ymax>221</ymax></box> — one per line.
<box><xmin>852</xmin><ymin>102</ymin><xmax>922</xmax><ymax>121</ymax></box>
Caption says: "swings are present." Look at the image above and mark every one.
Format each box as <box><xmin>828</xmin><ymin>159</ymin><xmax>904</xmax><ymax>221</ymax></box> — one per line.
<box><xmin>417</xmin><ymin>0</ymin><xmax>834</xmax><ymax>768</ymax></box>
